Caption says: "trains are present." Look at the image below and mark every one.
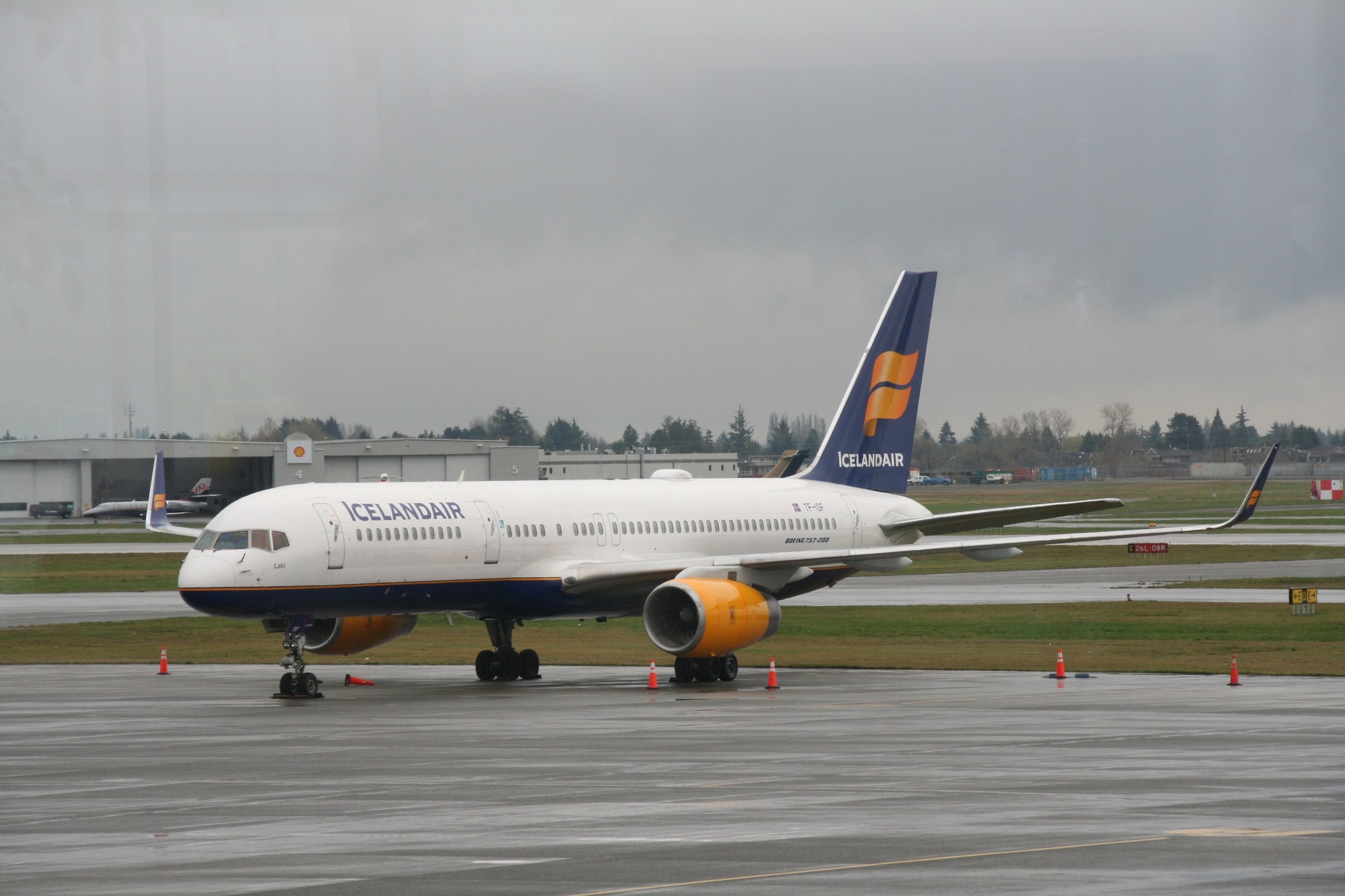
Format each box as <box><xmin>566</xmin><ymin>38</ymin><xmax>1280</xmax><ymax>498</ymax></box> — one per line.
<box><xmin>919</xmin><ymin>466</ymin><xmax>1097</xmax><ymax>482</ymax></box>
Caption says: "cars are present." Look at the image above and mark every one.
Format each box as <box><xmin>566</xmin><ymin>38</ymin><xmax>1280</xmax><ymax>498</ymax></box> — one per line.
<box><xmin>906</xmin><ymin>468</ymin><xmax>951</xmax><ymax>487</ymax></box>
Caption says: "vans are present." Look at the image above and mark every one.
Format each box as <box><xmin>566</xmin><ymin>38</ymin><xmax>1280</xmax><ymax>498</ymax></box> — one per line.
<box><xmin>28</xmin><ymin>503</ymin><xmax>73</xmax><ymax>519</ymax></box>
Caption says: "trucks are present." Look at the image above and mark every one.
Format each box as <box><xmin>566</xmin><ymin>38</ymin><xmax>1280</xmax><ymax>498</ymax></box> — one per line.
<box><xmin>968</xmin><ymin>474</ymin><xmax>1002</xmax><ymax>484</ymax></box>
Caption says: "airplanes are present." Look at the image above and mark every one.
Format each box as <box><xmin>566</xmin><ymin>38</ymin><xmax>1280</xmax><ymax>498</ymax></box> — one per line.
<box><xmin>144</xmin><ymin>270</ymin><xmax>1284</xmax><ymax>700</ymax></box>
<box><xmin>82</xmin><ymin>478</ymin><xmax>223</xmax><ymax>520</ymax></box>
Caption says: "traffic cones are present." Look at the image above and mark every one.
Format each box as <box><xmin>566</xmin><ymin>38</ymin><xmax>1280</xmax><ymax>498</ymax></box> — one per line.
<box><xmin>1226</xmin><ymin>655</ymin><xmax>1243</xmax><ymax>686</ymax></box>
<box><xmin>156</xmin><ymin>647</ymin><xmax>171</xmax><ymax>675</ymax></box>
<box><xmin>344</xmin><ymin>674</ymin><xmax>375</xmax><ymax>686</ymax></box>
<box><xmin>1054</xmin><ymin>648</ymin><xmax>1067</xmax><ymax>679</ymax></box>
<box><xmin>765</xmin><ymin>658</ymin><xmax>781</xmax><ymax>689</ymax></box>
<box><xmin>646</xmin><ymin>658</ymin><xmax>660</xmax><ymax>690</ymax></box>
